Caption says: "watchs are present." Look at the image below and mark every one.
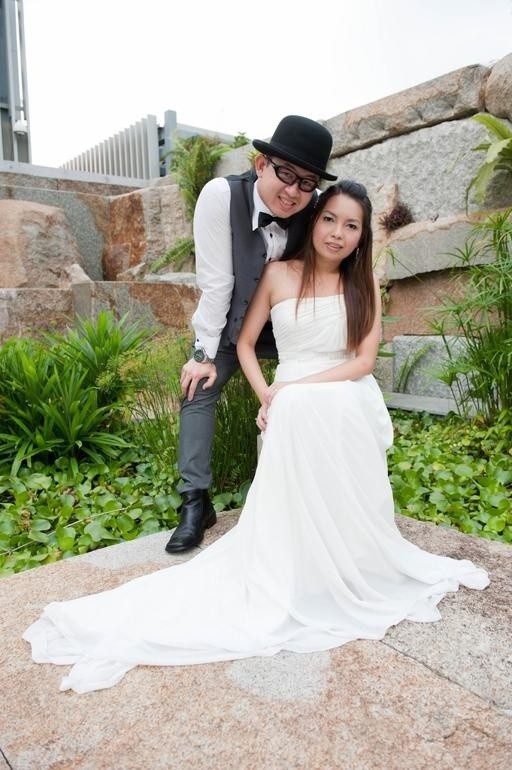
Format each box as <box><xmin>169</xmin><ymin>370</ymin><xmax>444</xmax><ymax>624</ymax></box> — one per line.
<box><xmin>192</xmin><ymin>346</ymin><xmax>214</xmax><ymax>364</ymax></box>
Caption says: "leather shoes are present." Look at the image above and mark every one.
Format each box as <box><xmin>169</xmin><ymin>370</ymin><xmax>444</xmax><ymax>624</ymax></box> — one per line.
<box><xmin>164</xmin><ymin>488</ymin><xmax>216</xmax><ymax>553</ymax></box>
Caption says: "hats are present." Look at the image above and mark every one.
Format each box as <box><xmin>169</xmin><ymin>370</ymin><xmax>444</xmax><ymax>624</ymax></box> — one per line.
<box><xmin>252</xmin><ymin>114</ymin><xmax>338</xmax><ymax>181</ymax></box>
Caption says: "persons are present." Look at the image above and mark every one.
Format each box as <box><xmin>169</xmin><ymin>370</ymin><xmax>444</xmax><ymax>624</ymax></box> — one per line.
<box><xmin>232</xmin><ymin>181</ymin><xmax>396</xmax><ymax>653</ymax></box>
<box><xmin>163</xmin><ymin>114</ymin><xmax>339</xmax><ymax>555</ymax></box>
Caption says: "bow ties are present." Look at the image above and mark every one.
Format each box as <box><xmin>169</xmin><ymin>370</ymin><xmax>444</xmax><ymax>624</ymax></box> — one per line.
<box><xmin>257</xmin><ymin>212</ymin><xmax>291</xmax><ymax>231</ymax></box>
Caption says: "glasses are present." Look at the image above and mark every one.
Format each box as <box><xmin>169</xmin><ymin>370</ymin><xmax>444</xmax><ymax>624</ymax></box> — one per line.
<box><xmin>269</xmin><ymin>158</ymin><xmax>319</xmax><ymax>193</ymax></box>
